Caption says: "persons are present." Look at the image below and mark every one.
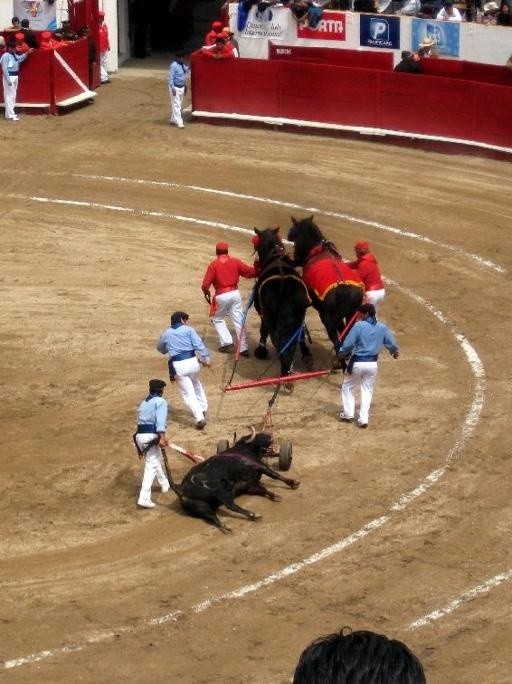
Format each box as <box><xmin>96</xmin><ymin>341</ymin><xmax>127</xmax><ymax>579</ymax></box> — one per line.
<box><xmin>168</xmin><ymin>51</ymin><xmax>191</xmax><ymax>128</ymax></box>
<box><xmin>156</xmin><ymin>311</ymin><xmax>212</xmax><ymax>430</ymax></box>
<box><xmin>201</xmin><ymin>242</ymin><xmax>256</xmax><ymax>357</ymax></box>
<box><xmin>337</xmin><ymin>303</ymin><xmax>400</xmax><ymax>428</ymax></box>
<box><xmin>132</xmin><ymin>379</ymin><xmax>170</xmax><ymax>508</ymax></box>
<box><xmin>292</xmin><ymin>625</ymin><xmax>426</xmax><ymax>684</ymax></box>
<box><xmin>0</xmin><ymin>11</ymin><xmax>111</xmax><ymax>121</ymax></box>
<box><xmin>343</xmin><ymin>240</ymin><xmax>385</xmax><ymax>319</ymax></box>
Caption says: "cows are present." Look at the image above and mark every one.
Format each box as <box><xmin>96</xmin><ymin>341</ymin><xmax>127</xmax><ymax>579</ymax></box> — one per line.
<box><xmin>158</xmin><ymin>422</ymin><xmax>301</xmax><ymax>536</ymax></box>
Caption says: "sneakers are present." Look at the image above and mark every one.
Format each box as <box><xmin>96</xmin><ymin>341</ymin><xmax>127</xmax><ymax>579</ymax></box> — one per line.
<box><xmin>219</xmin><ymin>343</ymin><xmax>234</xmax><ymax>351</ymax></box>
<box><xmin>340</xmin><ymin>411</ymin><xmax>354</xmax><ymax>419</ymax></box>
<box><xmin>138</xmin><ymin>500</ymin><xmax>155</xmax><ymax>507</ymax></box>
<box><xmin>196</xmin><ymin>419</ymin><xmax>205</xmax><ymax>428</ymax></box>
<box><xmin>241</xmin><ymin>350</ymin><xmax>248</xmax><ymax>357</ymax></box>
<box><xmin>358</xmin><ymin>419</ymin><xmax>368</xmax><ymax>428</ymax></box>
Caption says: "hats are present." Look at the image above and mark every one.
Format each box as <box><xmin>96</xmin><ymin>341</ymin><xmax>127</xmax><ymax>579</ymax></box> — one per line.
<box><xmin>15</xmin><ymin>31</ymin><xmax>53</xmax><ymax>41</ymax></box>
<box><xmin>418</xmin><ymin>37</ymin><xmax>435</xmax><ymax>47</ymax></box>
<box><xmin>353</xmin><ymin>240</ymin><xmax>369</xmax><ymax>250</ymax></box>
<box><xmin>359</xmin><ymin>303</ymin><xmax>376</xmax><ymax>314</ymax></box>
<box><xmin>211</xmin><ymin>21</ymin><xmax>234</xmax><ymax>40</ymax></box>
<box><xmin>215</xmin><ymin>242</ymin><xmax>229</xmax><ymax>250</ymax></box>
<box><xmin>149</xmin><ymin>379</ymin><xmax>166</xmax><ymax>388</ymax></box>
<box><xmin>171</xmin><ymin>311</ymin><xmax>189</xmax><ymax>320</ymax></box>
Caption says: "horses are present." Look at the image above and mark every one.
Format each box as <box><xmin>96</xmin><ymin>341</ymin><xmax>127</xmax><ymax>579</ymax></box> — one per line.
<box><xmin>252</xmin><ymin>225</ymin><xmax>315</xmax><ymax>394</ymax></box>
<box><xmin>287</xmin><ymin>215</ymin><xmax>367</xmax><ymax>372</ymax></box>
